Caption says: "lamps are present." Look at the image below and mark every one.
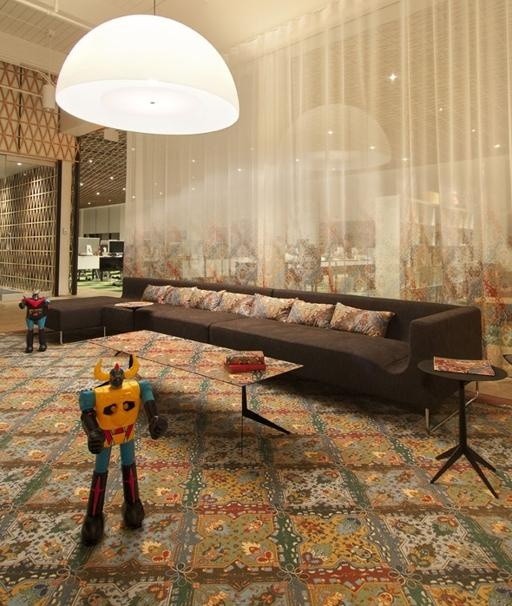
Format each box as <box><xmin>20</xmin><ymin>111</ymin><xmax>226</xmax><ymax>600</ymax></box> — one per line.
<box><xmin>55</xmin><ymin>0</ymin><xmax>240</xmax><ymax>135</ymax></box>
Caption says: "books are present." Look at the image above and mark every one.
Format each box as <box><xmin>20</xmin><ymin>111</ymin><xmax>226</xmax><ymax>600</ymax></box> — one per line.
<box><xmin>221</xmin><ymin>361</ymin><xmax>267</xmax><ymax>371</ymax></box>
<box><xmin>224</xmin><ymin>351</ymin><xmax>265</xmax><ymax>366</ymax></box>
<box><xmin>433</xmin><ymin>356</ymin><xmax>496</xmax><ymax>378</ymax></box>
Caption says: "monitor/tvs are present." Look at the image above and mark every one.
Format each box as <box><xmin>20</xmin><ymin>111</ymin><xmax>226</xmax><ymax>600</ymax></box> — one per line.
<box><xmin>108</xmin><ymin>240</ymin><xmax>124</xmax><ymax>257</ymax></box>
<box><xmin>78</xmin><ymin>237</ymin><xmax>100</xmax><ymax>256</ymax></box>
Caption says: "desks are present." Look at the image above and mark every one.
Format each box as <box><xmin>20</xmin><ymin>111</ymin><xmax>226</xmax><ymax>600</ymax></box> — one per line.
<box><xmin>417</xmin><ymin>359</ymin><xmax>508</xmax><ymax>499</ymax></box>
<box><xmin>114</xmin><ymin>302</ymin><xmax>155</xmax><ymax>357</ymax></box>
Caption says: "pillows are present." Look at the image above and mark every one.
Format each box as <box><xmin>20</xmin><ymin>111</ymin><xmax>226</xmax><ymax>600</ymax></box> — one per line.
<box><xmin>141</xmin><ymin>284</ymin><xmax>395</xmax><ymax>337</ymax></box>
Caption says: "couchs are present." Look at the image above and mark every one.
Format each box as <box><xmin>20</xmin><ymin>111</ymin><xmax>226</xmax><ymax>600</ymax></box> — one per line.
<box><xmin>43</xmin><ymin>274</ymin><xmax>482</xmax><ymax>433</ymax></box>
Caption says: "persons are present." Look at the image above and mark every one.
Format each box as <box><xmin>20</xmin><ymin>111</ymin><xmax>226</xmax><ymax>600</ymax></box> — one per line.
<box><xmin>75</xmin><ymin>353</ymin><xmax>168</xmax><ymax>548</ymax></box>
<box><xmin>17</xmin><ymin>289</ymin><xmax>52</xmax><ymax>353</ymax></box>
